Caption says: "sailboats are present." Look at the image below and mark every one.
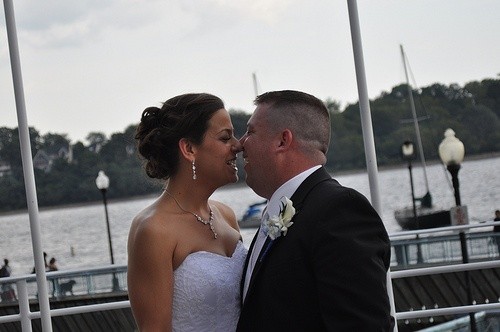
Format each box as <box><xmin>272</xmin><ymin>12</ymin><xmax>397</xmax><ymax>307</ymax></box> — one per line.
<box><xmin>394</xmin><ymin>42</ymin><xmax>470</xmax><ymax>229</ymax></box>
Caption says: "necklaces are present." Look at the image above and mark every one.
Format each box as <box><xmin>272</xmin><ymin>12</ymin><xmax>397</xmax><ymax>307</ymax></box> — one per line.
<box><xmin>165</xmin><ymin>189</ymin><xmax>219</xmax><ymax>240</ymax></box>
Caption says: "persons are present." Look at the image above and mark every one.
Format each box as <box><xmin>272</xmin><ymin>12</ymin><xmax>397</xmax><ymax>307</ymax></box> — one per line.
<box><xmin>0</xmin><ymin>259</ymin><xmax>18</xmax><ymax>303</ymax></box>
<box><xmin>46</xmin><ymin>258</ymin><xmax>59</xmax><ymax>296</ymax></box>
<box><xmin>492</xmin><ymin>210</ymin><xmax>500</xmax><ymax>259</ymax></box>
<box><xmin>33</xmin><ymin>252</ymin><xmax>48</xmax><ymax>273</ymax></box>
<box><xmin>127</xmin><ymin>93</ymin><xmax>249</xmax><ymax>332</ymax></box>
<box><xmin>240</xmin><ymin>90</ymin><xmax>395</xmax><ymax>332</ymax></box>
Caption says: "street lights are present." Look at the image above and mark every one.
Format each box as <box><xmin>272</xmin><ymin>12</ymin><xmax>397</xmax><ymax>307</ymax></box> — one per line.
<box><xmin>400</xmin><ymin>138</ymin><xmax>420</xmax><ymax>229</ymax></box>
<box><xmin>95</xmin><ymin>170</ymin><xmax>121</xmax><ymax>292</ymax></box>
<box><xmin>438</xmin><ymin>128</ymin><xmax>478</xmax><ymax>332</ymax></box>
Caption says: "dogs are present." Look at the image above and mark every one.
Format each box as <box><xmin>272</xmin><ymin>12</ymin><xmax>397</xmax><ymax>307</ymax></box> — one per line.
<box><xmin>58</xmin><ymin>279</ymin><xmax>76</xmax><ymax>297</ymax></box>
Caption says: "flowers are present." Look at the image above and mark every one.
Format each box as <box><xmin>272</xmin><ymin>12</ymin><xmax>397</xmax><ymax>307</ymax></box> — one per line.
<box><xmin>261</xmin><ymin>196</ymin><xmax>296</xmax><ymax>240</ymax></box>
